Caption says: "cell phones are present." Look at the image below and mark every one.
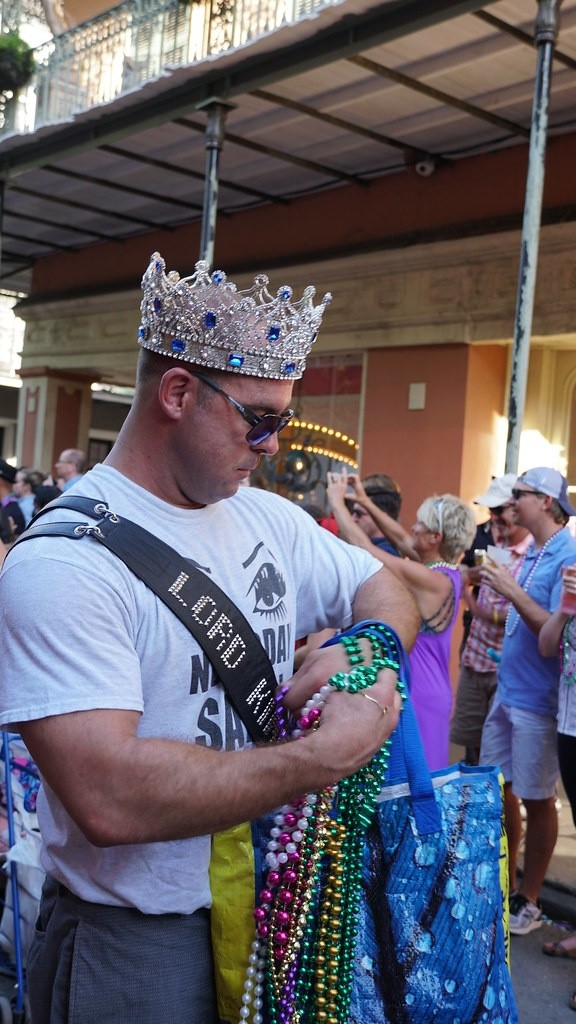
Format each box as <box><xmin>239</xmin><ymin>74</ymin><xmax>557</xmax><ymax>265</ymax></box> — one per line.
<box><xmin>560</xmin><ymin>566</ymin><xmax>575</xmax><ymax>616</ymax></box>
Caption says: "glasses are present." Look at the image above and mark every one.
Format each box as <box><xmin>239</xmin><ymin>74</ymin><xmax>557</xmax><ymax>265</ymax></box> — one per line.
<box><xmin>512</xmin><ymin>488</ymin><xmax>542</xmax><ymax>500</ymax></box>
<box><xmin>189</xmin><ymin>369</ymin><xmax>294</xmax><ymax>445</ymax></box>
<box><xmin>488</xmin><ymin>504</ymin><xmax>515</xmax><ymax>514</ymax></box>
<box><xmin>353</xmin><ymin>509</ymin><xmax>370</xmax><ymax>518</ymax></box>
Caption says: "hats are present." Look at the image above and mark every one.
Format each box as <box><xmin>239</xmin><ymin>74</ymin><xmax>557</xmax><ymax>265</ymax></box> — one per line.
<box><xmin>518</xmin><ymin>468</ymin><xmax>576</xmax><ymax>516</ymax></box>
<box><xmin>473</xmin><ymin>474</ymin><xmax>518</xmax><ymax>508</ymax></box>
<box><xmin>33</xmin><ymin>485</ymin><xmax>62</xmax><ymax>507</ymax></box>
<box><xmin>0</xmin><ymin>459</ymin><xmax>17</xmax><ymax>483</ymax></box>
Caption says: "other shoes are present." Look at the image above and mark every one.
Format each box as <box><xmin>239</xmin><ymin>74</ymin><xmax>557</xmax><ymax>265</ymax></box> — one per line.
<box><xmin>1</xmin><ymin>953</ymin><xmax>26</xmax><ymax>978</ymax></box>
<box><xmin>570</xmin><ymin>990</ymin><xmax>575</xmax><ymax>1011</ymax></box>
<box><xmin>543</xmin><ymin>940</ymin><xmax>576</xmax><ymax>958</ymax></box>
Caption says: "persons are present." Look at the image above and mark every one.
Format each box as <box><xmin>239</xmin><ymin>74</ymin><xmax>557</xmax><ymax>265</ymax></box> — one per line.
<box><xmin>0</xmin><ymin>441</ymin><xmax>574</xmax><ymax>958</ymax></box>
<box><xmin>0</xmin><ymin>270</ymin><xmax>419</xmax><ymax>1020</ymax></box>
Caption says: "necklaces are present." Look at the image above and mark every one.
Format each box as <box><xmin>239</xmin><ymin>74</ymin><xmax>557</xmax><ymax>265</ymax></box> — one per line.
<box><xmin>238</xmin><ymin>625</ymin><xmax>407</xmax><ymax>1023</ymax></box>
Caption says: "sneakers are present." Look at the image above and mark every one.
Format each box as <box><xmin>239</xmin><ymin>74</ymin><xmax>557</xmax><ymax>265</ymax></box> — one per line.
<box><xmin>509</xmin><ymin>893</ymin><xmax>542</xmax><ymax>936</ymax></box>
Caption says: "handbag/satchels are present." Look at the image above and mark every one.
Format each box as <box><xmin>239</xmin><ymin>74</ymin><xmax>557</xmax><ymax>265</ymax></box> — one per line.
<box><xmin>209</xmin><ymin>619</ymin><xmax>515</xmax><ymax>1022</ymax></box>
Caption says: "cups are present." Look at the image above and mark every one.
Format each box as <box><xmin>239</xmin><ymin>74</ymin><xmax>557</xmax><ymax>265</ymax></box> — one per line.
<box><xmin>483</xmin><ymin>544</ymin><xmax>512</xmax><ymax>578</ymax></box>
<box><xmin>561</xmin><ymin>562</ymin><xmax>576</xmax><ymax>616</ymax></box>
